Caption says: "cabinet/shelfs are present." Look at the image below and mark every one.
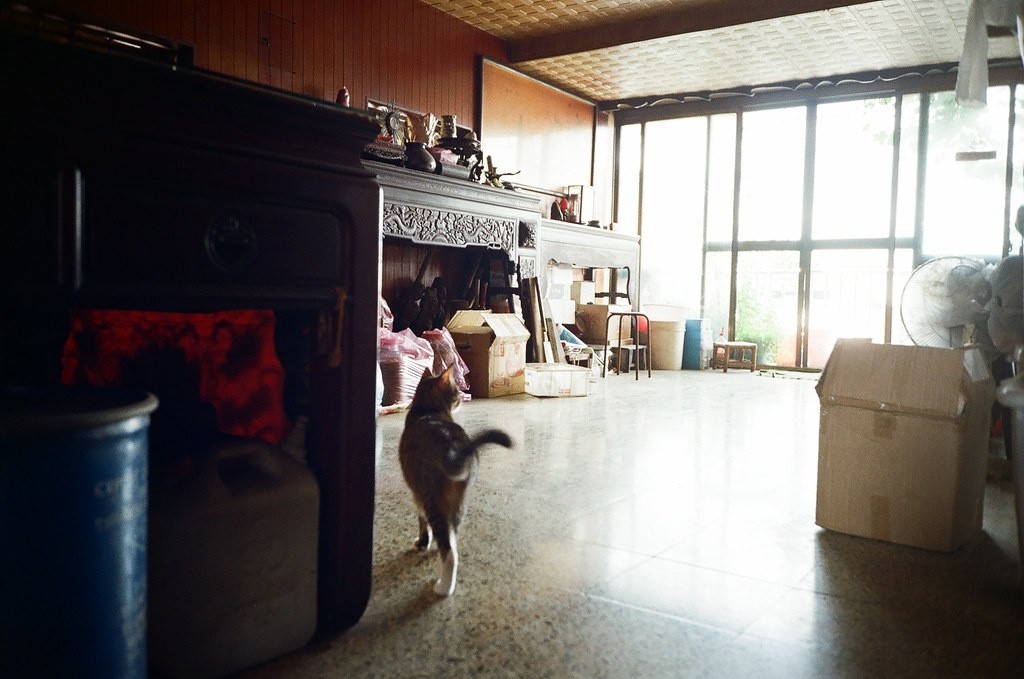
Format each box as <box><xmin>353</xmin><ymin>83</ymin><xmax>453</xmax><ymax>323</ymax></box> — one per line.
<box><xmin>0</xmin><ymin>0</ymin><xmax>378</xmax><ymax>632</ymax></box>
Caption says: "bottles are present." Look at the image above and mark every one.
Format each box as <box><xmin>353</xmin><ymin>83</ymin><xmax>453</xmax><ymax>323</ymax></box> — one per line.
<box><xmin>149</xmin><ymin>416</ymin><xmax>320</xmax><ymax>679</ymax></box>
<box><xmin>715</xmin><ymin>327</ymin><xmax>726</xmax><ymax>353</ymax></box>
<box><xmin>405</xmin><ymin>141</ymin><xmax>436</xmax><ymax>173</ymax></box>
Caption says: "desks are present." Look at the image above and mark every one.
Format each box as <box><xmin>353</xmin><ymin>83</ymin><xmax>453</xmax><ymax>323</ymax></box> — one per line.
<box><xmin>361</xmin><ymin>158</ymin><xmax>641</xmax><ymax>363</ymax></box>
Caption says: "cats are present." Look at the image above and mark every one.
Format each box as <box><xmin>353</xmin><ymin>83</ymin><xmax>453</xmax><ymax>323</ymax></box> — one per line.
<box><xmin>399</xmin><ymin>361</ymin><xmax>514</xmax><ymax>598</ymax></box>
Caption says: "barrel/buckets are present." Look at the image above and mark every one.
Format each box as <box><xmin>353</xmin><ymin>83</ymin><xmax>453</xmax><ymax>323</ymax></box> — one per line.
<box><xmin>0</xmin><ymin>386</ymin><xmax>160</xmax><ymax>678</ymax></box>
<box><xmin>649</xmin><ymin>320</ymin><xmax>684</xmax><ymax>370</ymax></box>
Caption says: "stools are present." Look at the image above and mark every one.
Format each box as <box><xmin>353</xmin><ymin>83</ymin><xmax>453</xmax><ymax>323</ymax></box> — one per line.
<box><xmin>713</xmin><ymin>341</ymin><xmax>757</xmax><ymax>372</ymax></box>
<box><xmin>615</xmin><ymin>345</ymin><xmax>648</xmax><ymax>373</ymax></box>
<box><xmin>603</xmin><ymin>313</ymin><xmax>652</xmax><ymax>380</ymax></box>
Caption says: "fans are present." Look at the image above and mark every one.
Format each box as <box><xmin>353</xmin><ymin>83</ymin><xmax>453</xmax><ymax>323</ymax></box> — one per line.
<box><xmin>900</xmin><ymin>256</ymin><xmax>1002</xmax><ymax>363</ymax></box>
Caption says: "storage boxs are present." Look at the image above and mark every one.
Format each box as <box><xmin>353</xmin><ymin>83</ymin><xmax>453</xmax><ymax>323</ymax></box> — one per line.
<box><xmin>587</xmin><ymin>338</ymin><xmax>633</xmax><ymax>370</ymax></box>
<box><xmin>526</xmin><ymin>363</ymin><xmax>592</xmax><ymax>397</ymax></box>
<box><xmin>568</xmin><ymin>185</ymin><xmax>595</xmax><ymax>224</ymax></box>
<box><xmin>815</xmin><ymin>339</ymin><xmax>997</xmax><ymax>551</ymax></box>
<box><xmin>446</xmin><ymin>310</ymin><xmax>531</xmax><ymax>396</ymax></box>
<box><xmin>577</xmin><ymin>304</ymin><xmax>631</xmax><ymax>339</ymax></box>
